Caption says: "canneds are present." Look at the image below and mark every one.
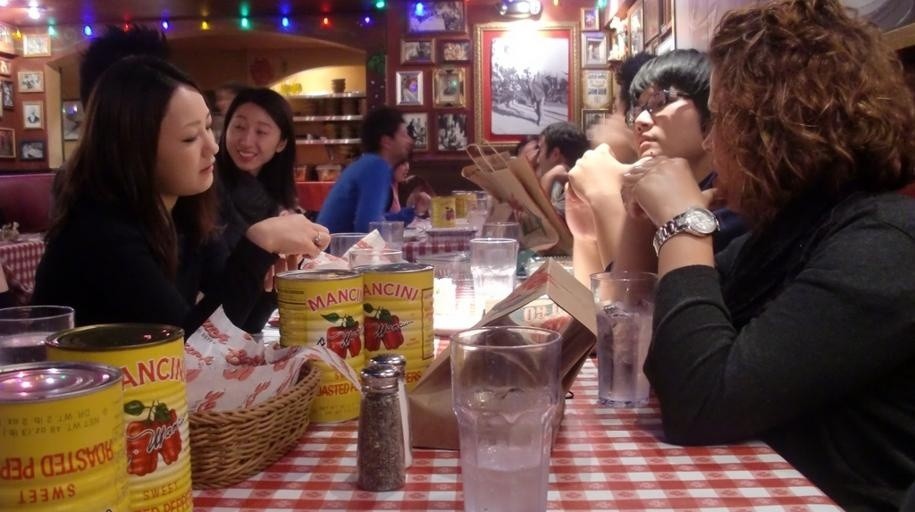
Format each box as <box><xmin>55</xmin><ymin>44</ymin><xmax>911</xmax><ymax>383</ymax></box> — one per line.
<box><xmin>452</xmin><ymin>190</ymin><xmax>469</xmax><ymax>218</ymax></box>
<box><xmin>469</xmin><ymin>190</ymin><xmax>478</xmax><ymax>212</ymax></box>
<box><xmin>1</xmin><ymin>361</ymin><xmax>130</xmax><ymax>512</ymax></box>
<box><xmin>276</xmin><ymin>268</ymin><xmax>363</xmax><ymax>427</ymax></box>
<box><xmin>362</xmin><ymin>262</ymin><xmax>434</xmax><ymax>399</ymax></box>
<box><xmin>45</xmin><ymin>322</ymin><xmax>193</xmax><ymax>512</ymax></box>
<box><xmin>431</xmin><ymin>195</ymin><xmax>457</xmax><ymax>227</ymax></box>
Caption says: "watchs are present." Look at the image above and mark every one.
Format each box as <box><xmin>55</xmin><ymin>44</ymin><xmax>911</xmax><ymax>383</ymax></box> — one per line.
<box><xmin>650</xmin><ymin>206</ymin><xmax>723</xmax><ymax>257</ymax></box>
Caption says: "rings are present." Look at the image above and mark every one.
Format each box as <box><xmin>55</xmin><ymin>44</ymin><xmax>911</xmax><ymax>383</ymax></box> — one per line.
<box><xmin>314</xmin><ymin>230</ymin><xmax>322</xmax><ymax>244</ymax></box>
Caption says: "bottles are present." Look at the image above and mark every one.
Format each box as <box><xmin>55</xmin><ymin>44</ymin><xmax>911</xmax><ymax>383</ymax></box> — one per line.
<box><xmin>357</xmin><ymin>354</ymin><xmax>416</xmax><ymax>491</ymax></box>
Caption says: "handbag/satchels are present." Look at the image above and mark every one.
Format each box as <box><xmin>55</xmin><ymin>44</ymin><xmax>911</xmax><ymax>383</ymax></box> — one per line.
<box><xmin>456</xmin><ymin>132</ymin><xmax>573</xmax><ymax>258</ymax></box>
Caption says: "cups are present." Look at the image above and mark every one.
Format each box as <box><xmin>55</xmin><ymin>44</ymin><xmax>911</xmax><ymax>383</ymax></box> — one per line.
<box><xmin>464</xmin><ymin>190</ymin><xmax>491</xmax><ymax>227</ymax></box>
<box><xmin>451</xmin><ymin>327</ymin><xmax>561</xmax><ymax>512</ymax></box>
<box><xmin>0</xmin><ymin>305</ymin><xmax>74</xmax><ymax>372</ymax></box>
<box><xmin>470</xmin><ymin>237</ymin><xmax>518</xmax><ymax>303</ymax></box>
<box><xmin>589</xmin><ymin>271</ymin><xmax>658</xmax><ymax>407</ymax></box>
<box><xmin>329</xmin><ymin>221</ymin><xmax>406</xmax><ymax>271</ymax></box>
<box><xmin>319</xmin><ymin>97</ymin><xmax>367</xmax><ymax>138</ymax></box>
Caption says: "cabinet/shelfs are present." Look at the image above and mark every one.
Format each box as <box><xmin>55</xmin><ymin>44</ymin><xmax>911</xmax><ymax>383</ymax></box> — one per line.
<box><xmin>283</xmin><ymin>91</ymin><xmax>368</xmax><ymax>145</ymax></box>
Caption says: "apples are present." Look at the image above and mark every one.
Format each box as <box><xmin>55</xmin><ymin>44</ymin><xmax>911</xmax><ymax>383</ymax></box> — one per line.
<box><xmin>364</xmin><ymin>304</ymin><xmax>404</xmax><ymax>351</ymax></box>
<box><xmin>321</xmin><ymin>312</ymin><xmax>361</xmax><ymax>358</ymax></box>
<box><xmin>125</xmin><ymin>401</ymin><xmax>182</xmax><ymax>476</ymax></box>
<box><xmin>446</xmin><ymin>207</ymin><xmax>453</xmax><ymax>220</ymax></box>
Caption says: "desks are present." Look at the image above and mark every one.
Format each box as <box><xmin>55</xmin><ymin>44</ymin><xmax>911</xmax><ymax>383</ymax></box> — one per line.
<box><xmin>400</xmin><ymin>196</ymin><xmax>519</xmax><ymax>259</ymax></box>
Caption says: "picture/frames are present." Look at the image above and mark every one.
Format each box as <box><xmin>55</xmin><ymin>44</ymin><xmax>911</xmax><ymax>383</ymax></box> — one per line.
<box><xmin>1</xmin><ymin>20</ymin><xmax>52</xmax><ymax>162</ymax></box>
<box><xmin>63</xmin><ymin>97</ymin><xmax>85</xmax><ymax>143</ymax></box>
<box><xmin>473</xmin><ymin>20</ymin><xmax>579</xmax><ymax>148</ymax></box>
<box><xmin>577</xmin><ymin>0</ymin><xmax>674</xmax><ymax>134</ymax></box>
<box><xmin>395</xmin><ymin>0</ymin><xmax>473</xmax><ymax>156</ymax></box>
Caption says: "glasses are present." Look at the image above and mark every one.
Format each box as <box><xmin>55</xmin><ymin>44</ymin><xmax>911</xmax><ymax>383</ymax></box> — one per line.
<box><xmin>624</xmin><ymin>87</ymin><xmax>698</xmax><ymax>130</ymax></box>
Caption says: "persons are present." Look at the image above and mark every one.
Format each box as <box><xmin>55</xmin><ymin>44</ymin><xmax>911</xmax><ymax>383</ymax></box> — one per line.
<box><xmin>400</xmin><ymin>73</ymin><xmax>420</xmax><ymax>102</ymax></box>
<box><xmin>26</xmin><ymin>107</ymin><xmax>40</xmax><ymax>126</ymax></box>
<box><xmin>212</xmin><ymin>87</ymin><xmax>303</xmax><ymax>227</ymax></box>
<box><xmin>385</xmin><ymin>152</ymin><xmax>411</xmax><ymax>213</ymax></box>
<box><xmin>436</xmin><ymin>113</ymin><xmax>466</xmax><ymax>150</ymax></box>
<box><xmin>397</xmin><ymin>175</ymin><xmax>432</xmax><ymax>210</ymax></box>
<box><xmin>49</xmin><ymin>20</ymin><xmax>169</xmax><ymax>228</ymax></box>
<box><xmin>2</xmin><ymin>85</ymin><xmax>14</xmax><ymax>107</ymax></box>
<box><xmin>312</xmin><ymin>104</ymin><xmax>432</xmax><ymax>260</ymax></box>
<box><xmin>27</xmin><ymin>145</ymin><xmax>36</xmax><ymax>157</ymax></box>
<box><xmin>599</xmin><ymin>1</ymin><xmax>914</xmax><ymax>512</ymax></box>
<box><xmin>209</xmin><ymin>82</ymin><xmax>238</xmax><ymax>144</ymax></box>
<box><xmin>404</xmin><ymin>115</ymin><xmax>427</xmax><ymax>148</ymax></box>
<box><xmin>26</xmin><ymin>54</ymin><xmax>331</xmax><ymax>349</ymax></box>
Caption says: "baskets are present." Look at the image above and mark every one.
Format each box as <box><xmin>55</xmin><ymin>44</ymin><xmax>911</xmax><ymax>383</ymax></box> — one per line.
<box><xmin>181</xmin><ymin>357</ymin><xmax>323</xmax><ymax>490</ymax></box>
